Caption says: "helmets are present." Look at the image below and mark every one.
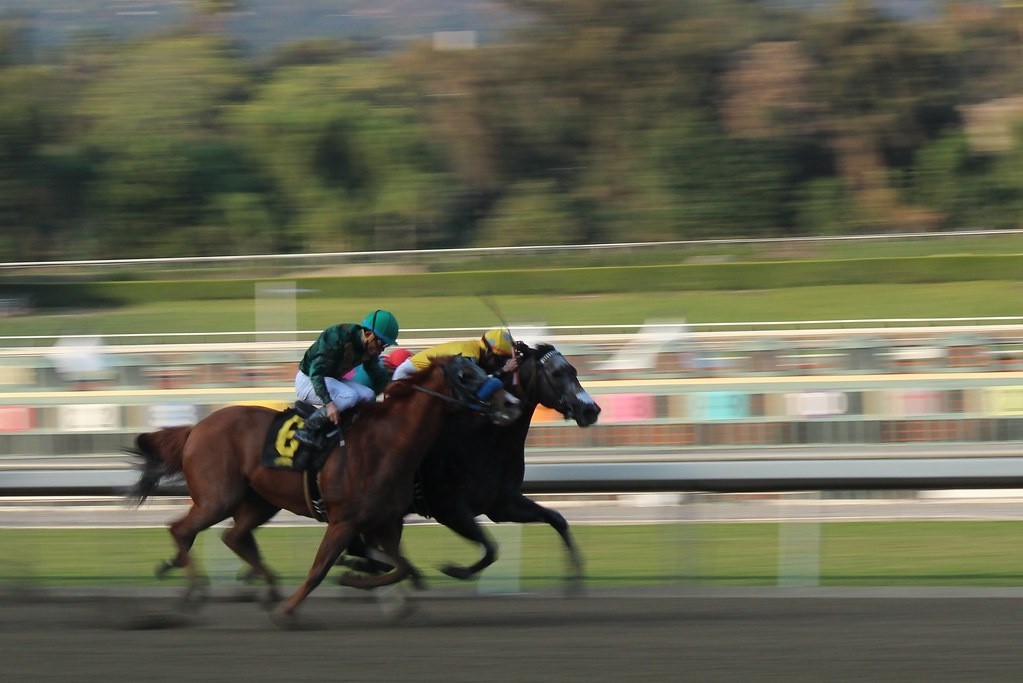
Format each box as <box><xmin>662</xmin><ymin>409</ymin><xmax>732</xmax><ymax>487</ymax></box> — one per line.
<box><xmin>479</xmin><ymin>329</ymin><xmax>515</xmax><ymax>355</ymax></box>
<box><xmin>360</xmin><ymin>310</ymin><xmax>400</xmax><ymax>346</ymax></box>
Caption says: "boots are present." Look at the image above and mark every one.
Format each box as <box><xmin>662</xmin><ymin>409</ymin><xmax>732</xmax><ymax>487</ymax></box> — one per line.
<box><xmin>294</xmin><ymin>408</ymin><xmax>332</xmax><ymax>448</ymax></box>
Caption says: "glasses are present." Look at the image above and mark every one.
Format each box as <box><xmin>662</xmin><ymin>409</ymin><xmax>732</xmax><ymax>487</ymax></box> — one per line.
<box><xmin>374</xmin><ymin>337</ymin><xmax>387</xmax><ymax>348</ymax></box>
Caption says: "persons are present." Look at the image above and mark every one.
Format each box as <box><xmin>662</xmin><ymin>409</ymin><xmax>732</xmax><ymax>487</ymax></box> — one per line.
<box><xmin>295</xmin><ymin>310</ymin><xmax>399</xmax><ymax>446</ymax></box>
<box><xmin>392</xmin><ymin>330</ymin><xmax>518</xmax><ymax>381</ymax></box>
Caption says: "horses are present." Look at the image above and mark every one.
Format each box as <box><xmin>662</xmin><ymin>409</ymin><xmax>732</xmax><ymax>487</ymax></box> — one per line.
<box><xmin>134</xmin><ymin>341</ymin><xmax>602</xmax><ymax>631</ymax></box>
